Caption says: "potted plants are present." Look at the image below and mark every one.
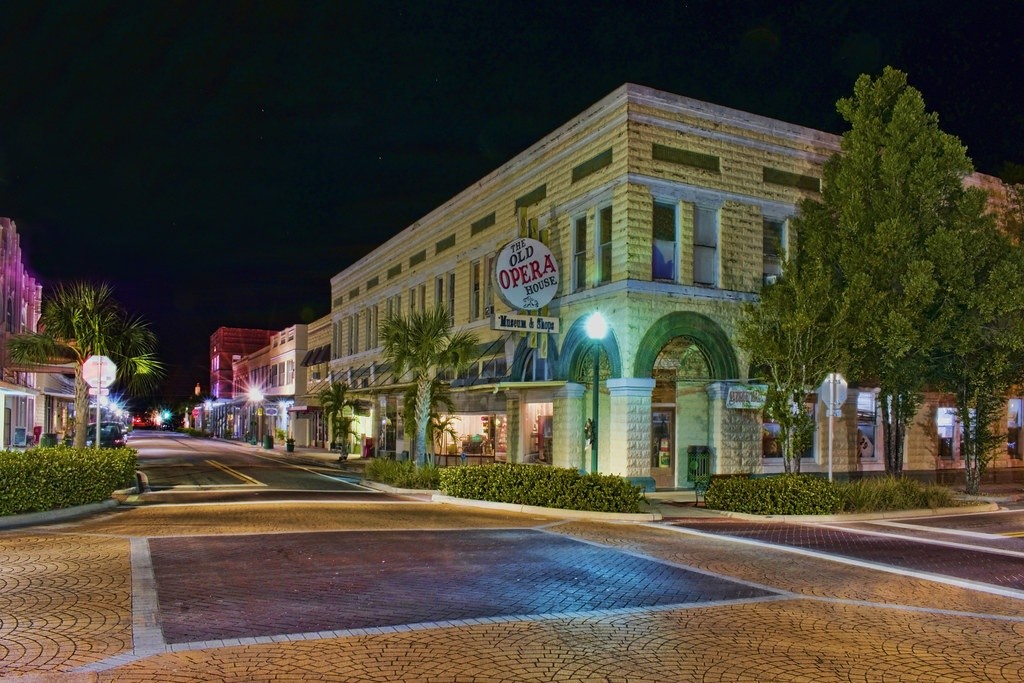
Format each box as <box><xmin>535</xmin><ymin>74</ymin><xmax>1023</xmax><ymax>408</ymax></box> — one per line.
<box><xmin>285</xmin><ymin>438</ymin><xmax>295</xmax><ymax>451</ymax></box>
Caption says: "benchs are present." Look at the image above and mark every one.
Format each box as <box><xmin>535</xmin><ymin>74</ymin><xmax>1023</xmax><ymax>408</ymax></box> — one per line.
<box><xmin>693</xmin><ymin>472</ymin><xmax>754</xmax><ymax>509</ymax></box>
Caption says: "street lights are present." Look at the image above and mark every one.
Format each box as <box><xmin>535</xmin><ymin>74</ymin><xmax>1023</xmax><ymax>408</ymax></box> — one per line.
<box><xmin>584</xmin><ymin>310</ymin><xmax>608</xmax><ymax>475</ymax></box>
<box><xmin>250</xmin><ymin>389</ymin><xmax>259</xmax><ymax>446</ymax></box>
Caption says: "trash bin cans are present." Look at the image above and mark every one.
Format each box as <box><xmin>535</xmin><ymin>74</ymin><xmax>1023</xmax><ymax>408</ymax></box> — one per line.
<box><xmin>263</xmin><ymin>435</ymin><xmax>273</xmax><ymax>449</ymax></box>
<box><xmin>40</xmin><ymin>433</ymin><xmax>59</xmax><ymax>448</ymax></box>
<box><xmin>686</xmin><ymin>444</ymin><xmax>711</xmax><ymax>491</ymax></box>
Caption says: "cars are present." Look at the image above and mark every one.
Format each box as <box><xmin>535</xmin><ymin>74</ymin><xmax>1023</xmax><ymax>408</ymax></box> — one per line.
<box><xmin>87</xmin><ymin>421</ymin><xmax>128</xmax><ymax>449</ymax></box>
